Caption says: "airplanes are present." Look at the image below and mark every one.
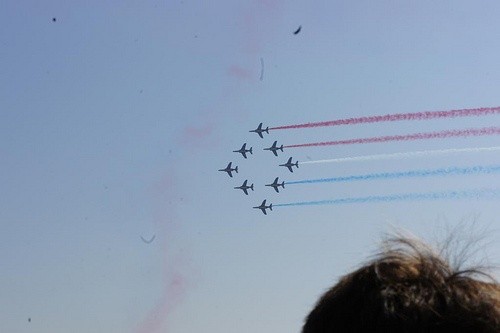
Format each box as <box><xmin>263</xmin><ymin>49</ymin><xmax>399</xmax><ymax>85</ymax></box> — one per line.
<box><xmin>265</xmin><ymin>177</ymin><xmax>285</xmax><ymax>193</ymax></box>
<box><xmin>234</xmin><ymin>179</ymin><xmax>254</xmax><ymax>195</ymax></box>
<box><xmin>264</xmin><ymin>141</ymin><xmax>284</xmax><ymax>157</ymax></box>
<box><xmin>218</xmin><ymin>162</ymin><xmax>238</xmax><ymax>178</ymax></box>
<box><xmin>253</xmin><ymin>199</ymin><xmax>273</xmax><ymax>216</ymax></box>
<box><xmin>232</xmin><ymin>143</ymin><xmax>253</xmax><ymax>159</ymax></box>
<box><xmin>278</xmin><ymin>157</ymin><xmax>298</xmax><ymax>174</ymax></box>
<box><xmin>250</xmin><ymin>123</ymin><xmax>269</xmax><ymax>139</ymax></box>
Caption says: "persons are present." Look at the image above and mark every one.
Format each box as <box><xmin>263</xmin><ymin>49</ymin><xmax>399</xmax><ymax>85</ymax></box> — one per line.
<box><xmin>300</xmin><ymin>227</ymin><xmax>500</xmax><ymax>333</ymax></box>
